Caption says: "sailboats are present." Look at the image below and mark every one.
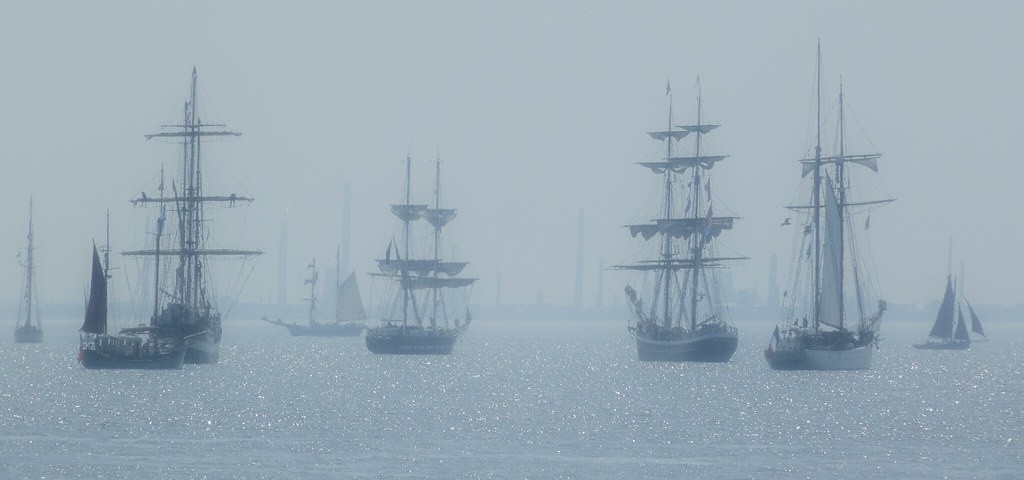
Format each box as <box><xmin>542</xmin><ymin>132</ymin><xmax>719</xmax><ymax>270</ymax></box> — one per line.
<box><xmin>11</xmin><ymin>193</ymin><xmax>45</xmax><ymax>344</ymax></box>
<box><xmin>120</xmin><ymin>62</ymin><xmax>265</xmax><ymax>372</ymax></box>
<box><xmin>76</xmin><ymin>210</ymin><xmax>186</xmax><ymax>371</ymax></box>
<box><xmin>259</xmin><ymin>244</ymin><xmax>366</xmax><ymax>337</ymax></box>
<box><xmin>762</xmin><ymin>35</ymin><xmax>885</xmax><ymax>369</ymax></box>
<box><xmin>603</xmin><ymin>71</ymin><xmax>750</xmax><ymax>363</ymax></box>
<box><xmin>911</xmin><ymin>234</ymin><xmax>988</xmax><ymax>352</ymax></box>
<box><xmin>363</xmin><ymin>147</ymin><xmax>479</xmax><ymax>357</ymax></box>
